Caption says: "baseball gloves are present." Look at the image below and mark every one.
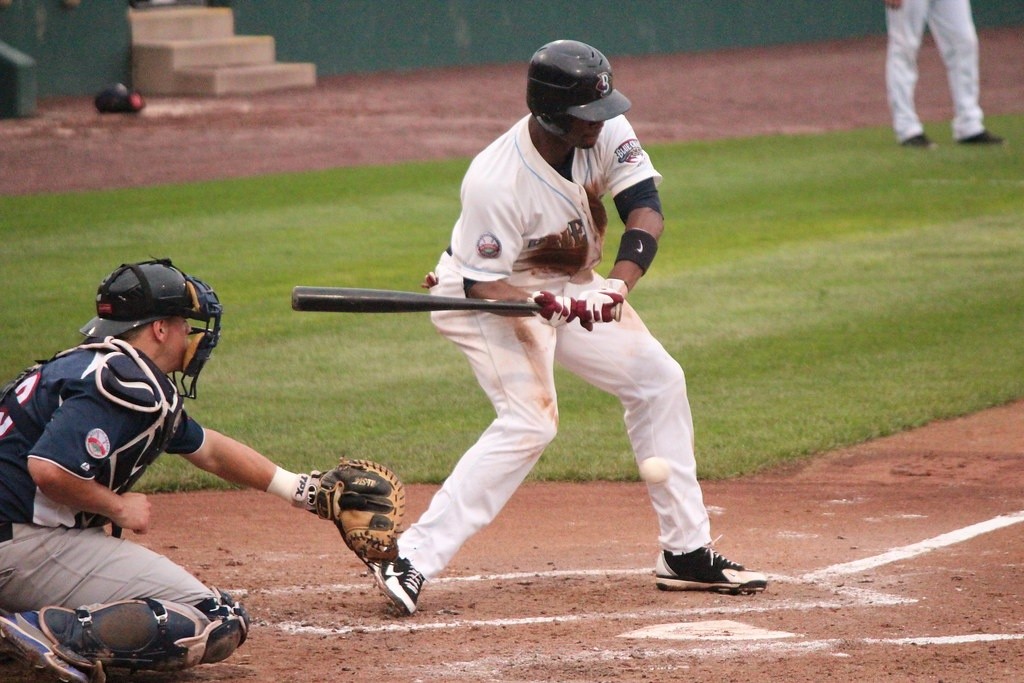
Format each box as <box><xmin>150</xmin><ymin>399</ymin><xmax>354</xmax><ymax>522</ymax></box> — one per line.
<box><xmin>309</xmin><ymin>459</ymin><xmax>408</xmax><ymax>563</ymax></box>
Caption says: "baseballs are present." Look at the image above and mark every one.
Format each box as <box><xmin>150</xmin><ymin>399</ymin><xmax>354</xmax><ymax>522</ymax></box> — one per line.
<box><xmin>637</xmin><ymin>455</ymin><xmax>673</xmax><ymax>489</ymax></box>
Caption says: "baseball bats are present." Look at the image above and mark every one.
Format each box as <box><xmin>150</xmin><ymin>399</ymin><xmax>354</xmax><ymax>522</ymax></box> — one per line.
<box><xmin>289</xmin><ymin>285</ymin><xmax>626</xmax><ymax>323</ymax></box>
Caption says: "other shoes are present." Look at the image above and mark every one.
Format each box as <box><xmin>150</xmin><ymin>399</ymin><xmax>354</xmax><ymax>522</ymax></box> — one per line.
<box><xmin>0</xmin><ymin>610</ymin><xmax>90</xmax><ymax>683</ymax></box>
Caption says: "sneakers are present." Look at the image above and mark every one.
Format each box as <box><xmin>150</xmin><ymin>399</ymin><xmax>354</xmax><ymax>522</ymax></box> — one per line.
<box><xmin>367</xmin><ymin>559</ymin><xmax>426</xmax><ymax>617</ymax></box>
<box><xmin>955</xmin><ymin>132</ymin><xmax>1005</xmax><ymax>147</ymax></box>
<box><xmin>655</xmin><ymin>534</ymin><xmax>767</xmax><ymax>593</ymax></box>
<box><xmin>899</xmin><ymin>132</ymin><xmax>938</xmax><ymax>149</ymax></box>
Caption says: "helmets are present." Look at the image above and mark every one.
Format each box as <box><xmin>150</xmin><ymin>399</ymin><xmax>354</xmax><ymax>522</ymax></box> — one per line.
<box><xmin>78</xmin><ymin>253</ymin><xmax>195</xmax><ymax>342</ymax></box>
<box><xmin>526</xmin><ymin>38</ymin><xmax>633</xmax><ymax>139</ymax></box>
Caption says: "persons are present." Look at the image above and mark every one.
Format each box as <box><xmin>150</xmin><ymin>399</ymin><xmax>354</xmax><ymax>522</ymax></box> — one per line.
<box><xmin>369</xmin><ymin>42</ymin><xmax>771</xmax><ymax>612</ymax></box>
<box><xmin>0</xmin><ymin>257</ymin><xmax>407</xmax><ymax>683</ymax></box>
<box><xmin>882</xmin><ymin>0</ymin><xmax>1007</xmax><ymax>148</ymax></box>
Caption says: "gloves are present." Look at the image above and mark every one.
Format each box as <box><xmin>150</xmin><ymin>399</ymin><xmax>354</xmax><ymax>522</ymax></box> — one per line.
<box><xmin>530</xmin><ymin>277</ymin><xmax>630</xmax><ymax>333</ymax></box>
